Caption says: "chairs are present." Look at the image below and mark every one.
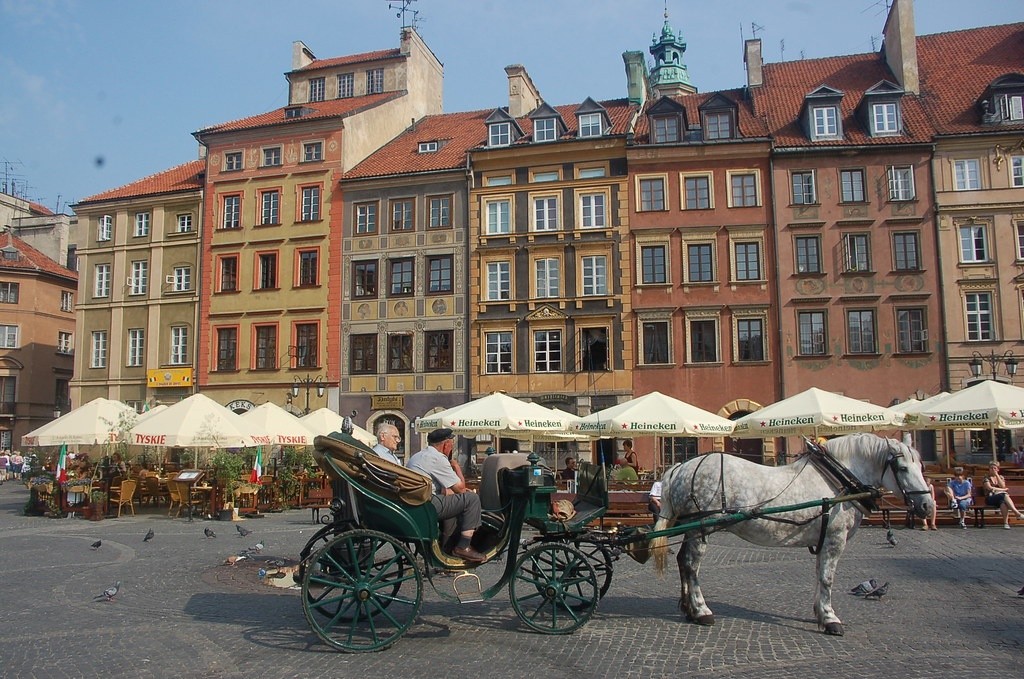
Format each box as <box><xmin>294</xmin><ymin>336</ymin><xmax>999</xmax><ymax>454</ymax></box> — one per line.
<box><xmin>89</xmin><ymin>472</ymin><xmax>274</xmax><ymax>518</ymax></box>
<box><xmin>560</xmin><ymin>473</ymin><xmax>572</xmax><ymax>484</ymax></box>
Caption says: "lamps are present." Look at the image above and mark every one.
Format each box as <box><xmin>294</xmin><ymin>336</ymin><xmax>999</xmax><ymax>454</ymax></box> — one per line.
<box><xmin>53</xmin><ymin>395</ymin><xmax>71</xmax><ymax>418</ymax></box>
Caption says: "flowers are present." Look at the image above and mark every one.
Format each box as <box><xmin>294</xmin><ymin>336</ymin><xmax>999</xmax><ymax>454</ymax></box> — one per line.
<box><xmin>90</xmin><ymin>491</ymin><xmax>107</xmax><ymax>503</ymax></box>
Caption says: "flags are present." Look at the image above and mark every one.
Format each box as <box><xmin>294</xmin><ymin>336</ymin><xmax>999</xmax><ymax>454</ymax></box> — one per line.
<box><xmin>55</xmin><ymin>441</ymin><xmax>66</xmax><ymax>484</ymax></box>
<box><xmin>249</xmin><ymin>444</ymin><xmax>262</xmax><ymax>486</ymax></box>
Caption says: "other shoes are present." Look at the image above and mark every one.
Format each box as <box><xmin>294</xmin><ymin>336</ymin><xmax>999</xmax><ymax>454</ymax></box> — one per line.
<box><xmin>1016</xmin><ymin>514</ymin><xmax>1024</xmax><ymax>520</ymax></box>
<box><xmin>922</xmin><ymin>524</ymin><xmax>938</xmax><ymax>531</ymax></box>
<box><xmin>959</xmin><ymin>519</ymin><xmax>967</xmax><ymax>530</ymax></box>
<box><xmin>1004</xmin><ymin>523</ymin><xmax>1011</xmax><ymax>530</ymax></box>
<box><xmin>951</xmin><ymin>500</ymin><xmax>958</xmax><ymax>508</ymax></box>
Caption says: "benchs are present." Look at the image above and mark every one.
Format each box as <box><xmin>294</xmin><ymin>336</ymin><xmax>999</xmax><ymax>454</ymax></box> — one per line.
<box><xmin>305</xmin><ymin>489</ymin><xmax>333</xmax><ymax>525</ymax></box>
<box><xmin>868</xmin><ymin>486</ymin><xmax>1024</xmax><ymax>530</ymax></box>
<box><xmin>552</xmin><ymin>493</ymin><xmax>654</xmax><ymax>527</ymax></box>
<box><xmin>925</xmin><ymin>464</ymin><xmax>955</xmax><ymax>474</ymax></box>
<box><xmin>328</xmin><ymin>431</ymin><xmax>440</xmax><ymax>541</ymax></box>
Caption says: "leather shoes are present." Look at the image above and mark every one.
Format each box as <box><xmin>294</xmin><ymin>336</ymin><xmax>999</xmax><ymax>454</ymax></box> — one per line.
<box><xmin>453</xmin><ymin>546</ymin><xmax>487</xmax><ymax>562</ymax></box>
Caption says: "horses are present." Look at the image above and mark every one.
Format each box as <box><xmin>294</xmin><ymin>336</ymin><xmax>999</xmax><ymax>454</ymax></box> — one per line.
<box><xmin>650</xmin><ymin>433</ymin><xmax>933</xmax><ymax>637</ymax></box>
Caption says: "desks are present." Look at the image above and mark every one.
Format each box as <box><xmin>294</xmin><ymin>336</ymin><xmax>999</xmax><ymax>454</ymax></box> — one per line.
<box><xmin>925</xmin><ymin>474</ymin><xmax>955</xmax><ymax>509</ymax></box>
<box><xmin>637</xmin><ymin>469</ymin><xmax>659</xmax><ymax>482</ymax></box>
<box><xmin>964</xmin><ymin>464</ymin><xmax>1016</xmax><ymax>468</ymax></box>
<box><xmin>190</xmin><ymin>486</ymin><xmax>212</xmax><ymax>517</ymax></box>
<box><xmin>141</xmin><ymin>478</ymin><xmax>169</xmax><ymax>483</ymax></box>
<box><xmin>1004</xmin><ymin>469</ymin><xmax>1024</xmax><ymax>471</ymax></box>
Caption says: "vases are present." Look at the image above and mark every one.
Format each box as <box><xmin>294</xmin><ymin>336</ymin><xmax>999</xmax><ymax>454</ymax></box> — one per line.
<box><xmin>90</xmin><ymin>503</ymin><xmax>104</xmax><ymax>520</ymax></box>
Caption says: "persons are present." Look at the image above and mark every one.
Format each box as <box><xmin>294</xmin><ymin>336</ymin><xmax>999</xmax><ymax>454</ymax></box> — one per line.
<box><xmin>1010</xmin><ymin>447</ymin><xmax>1019</xmax><ymax>463</ymax></box>
<box><xmin>648</xmin><ymin>466</ymin><xmax>674</xmax><ymax>523</ymax></box>
<box><xmin>944</xmin><ymin>467</ymin><xmax>972</xmax><ymax>527</ymax></box>
<box><xmin>406</xmin><ymin>428</ymin><xmax>486</xmax><ymax>563</ymax></box>
<box><xmin>982</xmin><ymin>461</ymin><xmax>1024</xmax><ymax>529</ymax></box>
<box><xmin>922</xmin><ymin>475</ymin><xmax>938</xmax><ymax>531</ymax></box>
<box><xmin>372</xmin><ymin>423</ymin><xmax>401</xmax><ymax>466</ymax></box>
<box><xmin>1017</xmin><ymin>445</ymin><xmax>1024</xmax><ymax>463</ymax></box>
<box><xmin>0</xmin><ymin>449</ymin><xmax>38</xmax><ymax>485</ymax></box>
<box><xmin>614</xmin><ymin>439</ymin><xmax>640</xmax><ymax>491</ymax></box>
<box><xmin>561</xmin><ymin>457</ymin><xmax>579</xmax><ymax>490</ymax></box>
<box><xmin>109</xmin><ymin>452</ymin><xmax>126</xmax><ymax>481</ymax></box>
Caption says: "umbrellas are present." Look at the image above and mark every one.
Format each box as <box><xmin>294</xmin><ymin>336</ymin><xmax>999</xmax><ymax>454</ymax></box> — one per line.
<box><xmin>20</xmin><ymin>393</ymin><xmax>169</xmax><ymax>477</ymax></box>
<box><xmin>126</xmin><ymin>392</ymin><xmax>271</xmax><ymax>470</ymax></box>
<box><xmin>571</xmin><ymin>391</ymin><xmax>737</xmax><ymax>478</ymax></box>
<box><xmin>414</xmin><ymin>391</ymin><xmax>612</xmax><ymax>479</ymax></box>
<box><xmin>889</xmin><ymin>380</ymin><xmax>1024</xmax><ymax>470</ymax></box>
<box><xmin>239</xmin><ymin>401</ymin><xmax>379</xmax><ymax>469</ymax></box>
<box><xmin>731</xmin><ymin>386</ymin><xmax>906</xmax><ymax>444</ymax></box>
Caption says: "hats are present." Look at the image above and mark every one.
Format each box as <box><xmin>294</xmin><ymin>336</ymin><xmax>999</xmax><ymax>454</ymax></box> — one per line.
<box><xmin>427</xmin><ymin>428</ymin><xmax>456</xmax><ymax>443</ymax></box>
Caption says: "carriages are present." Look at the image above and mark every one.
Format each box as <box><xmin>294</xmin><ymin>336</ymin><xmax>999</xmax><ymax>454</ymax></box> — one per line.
<box><xmin>293</xmin><ymin>416</ymin><xmax>938</xmax><ymax>654</ymax></box>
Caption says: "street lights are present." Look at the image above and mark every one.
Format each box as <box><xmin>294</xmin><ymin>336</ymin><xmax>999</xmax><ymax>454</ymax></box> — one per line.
<box><xmin>967</xmin><ymin>348</ymin><xmax>1019</xmax><ymax>461</ymax></box>
<box><xmin>292</xmin><ymin>374</ymin><xmax>326</xmax><ymax>414</ymax></box>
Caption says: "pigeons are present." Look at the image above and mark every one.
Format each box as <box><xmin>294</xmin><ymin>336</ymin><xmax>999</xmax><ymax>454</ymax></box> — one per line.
<box><xmin>887</xmin><ymin>528</ymin><xmax>900</xmax><ymax>547</ymax></box>
<box><xmin>851</xmin><ymin>578</ymin><xmax>891</xmax><ymax>601</ymax></box>
<box><xmin>94</xmin><ymin>580</ymin><xmax>121</xmax><ymax>602</ymax></box>
<box><xmin>143</xmin><ymin>528</ymin><xmax>154</xmax><ymax>542</ymax></box>
<box><xmin>222</xmin><ymin>539</ymin><xmax>291</xmax><ymax>567</ymax></box>
<box><xmin>236</xmin><ymin>524</ymin><xmax>253</xmax><ymax>536</ymax></box>
<box><xmin>91</xmin><ymin>539</ymin><xmax>103</xmax><ymax>551</ymax></box>
<box><xmin>205</xmin><ymin>527</ymin><xmax>216</xmax><ymax>538</ymax></box>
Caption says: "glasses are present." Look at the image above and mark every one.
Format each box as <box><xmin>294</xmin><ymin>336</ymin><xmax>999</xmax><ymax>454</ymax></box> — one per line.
<box><xmin>389</xmin><ymin>435</ymin><xmax>402</xmax><ymax>441</ymax></box>
